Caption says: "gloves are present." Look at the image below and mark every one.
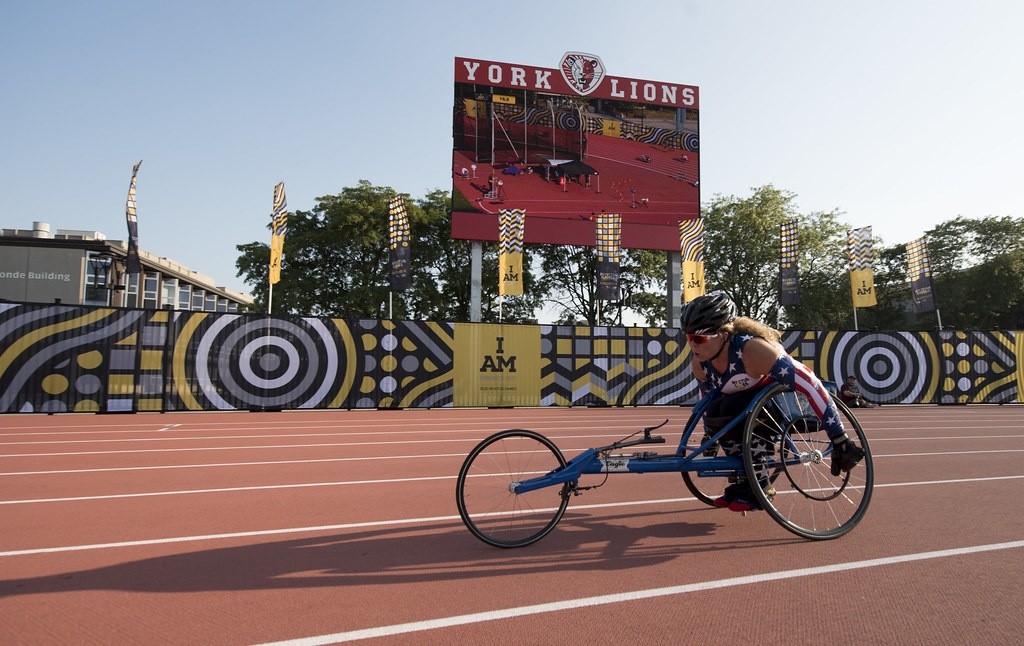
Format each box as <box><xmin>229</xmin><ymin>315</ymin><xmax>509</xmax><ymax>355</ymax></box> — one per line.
<box><xmin>701</xmin><ymin>433</ymin><xmax>719</xmax><ymax>457</ymax></box>
<box><xmin>829</xmin><ymin>432</ymin><xmax>866</xmax><ymax>477</ymax></box>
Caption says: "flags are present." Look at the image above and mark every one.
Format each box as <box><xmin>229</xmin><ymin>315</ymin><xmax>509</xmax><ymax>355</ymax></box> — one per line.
<box><xmin>125</xmin><ymin>159</ymin><xmax>143</xmax><ymax>274</ymax></box>
<box><xmin>498</xmin><ymin>207</ymin><xmax>527</xmax><ymax>298</ymax></box>
<box><xmin>594</xmin><ymin>213</ymin><xmax>622</xmax><ymax>300</ymax></box>
<box><xmin>848</xmin><ymin>225</ymin><xmax>879</xmax><ymax>307</ymax></box>
<box><xmin>268</xmin><ymin>180</ymin><xmax>288</xmax><ymax>284</ymax></box>
<box><xmin>677</xmin><ymin>218</ymin><xmax>706</xmax><ymax>303</ymax></box>
<box><xmin>780</xmin><ymin>218</ymin><xmax>801</xmax><ymax>306</ymax></box>
<box><xmin>388</xmin><ymin>193</ymin><xmax>412</xmax><ymax>290</ymax></box>
<box><xmin>905</xmin><ymin>233</ymin><xmax>940</xmax><ymax>313</ymax></box>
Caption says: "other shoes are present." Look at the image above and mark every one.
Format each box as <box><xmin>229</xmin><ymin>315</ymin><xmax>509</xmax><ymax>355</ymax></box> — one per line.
<box><xmin>867</xmin><ymin>403</ymin><xmax>878</xmax><ymax>408</ymax></box>
<box><xmin>725</xmin><ymin>481</ymin><xmax>777</xmax><ymax>504</ymax></box>
<box><xmin>854</xmin><ymin>401</ymin><xmax>860</xmax><ymax>408</ymax></box>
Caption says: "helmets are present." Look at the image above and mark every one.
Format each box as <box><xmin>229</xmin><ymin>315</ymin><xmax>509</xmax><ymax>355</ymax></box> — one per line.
<box><xmin>680</xmin><ymin>291</ymin><xmax>737</xmax><ymax>334</ymax></box>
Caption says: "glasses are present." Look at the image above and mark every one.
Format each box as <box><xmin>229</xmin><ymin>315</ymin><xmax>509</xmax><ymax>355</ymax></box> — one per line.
<box><xmin>687</xmin><ymin>334</ymin><xmax>718</xmax><ymax>345</ymax></box>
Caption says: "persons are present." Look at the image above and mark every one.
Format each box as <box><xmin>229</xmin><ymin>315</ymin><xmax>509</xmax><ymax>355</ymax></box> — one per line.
<box><xmin>841</xmin><ymin>375</ymin><xmax>879</xmax><ymax>408</ymax></box>
<box><xmin>680</xmin><ymin>295</ymin><xmax>866</xmax><ymax>515</ymax></box>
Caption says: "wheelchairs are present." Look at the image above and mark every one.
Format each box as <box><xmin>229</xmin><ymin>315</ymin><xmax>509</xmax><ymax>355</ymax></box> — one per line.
<box><xmin>455</xmin><ymin>372</ymin><xmax>875</xmax><ymax>549</ymax></box>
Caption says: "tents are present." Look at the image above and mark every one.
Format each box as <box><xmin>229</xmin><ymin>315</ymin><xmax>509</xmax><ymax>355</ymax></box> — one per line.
<box><xmin>547</xmin><ymin>158</ymin><xmax>599</xmax><ymax>193</ymax></box>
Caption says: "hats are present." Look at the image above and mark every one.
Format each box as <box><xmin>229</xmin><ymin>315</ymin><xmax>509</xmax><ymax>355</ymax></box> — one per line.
<box><xmin>847</xmin><ymin>376</ymin><xmax>857</xmax><ymax>380</ymax></box>
